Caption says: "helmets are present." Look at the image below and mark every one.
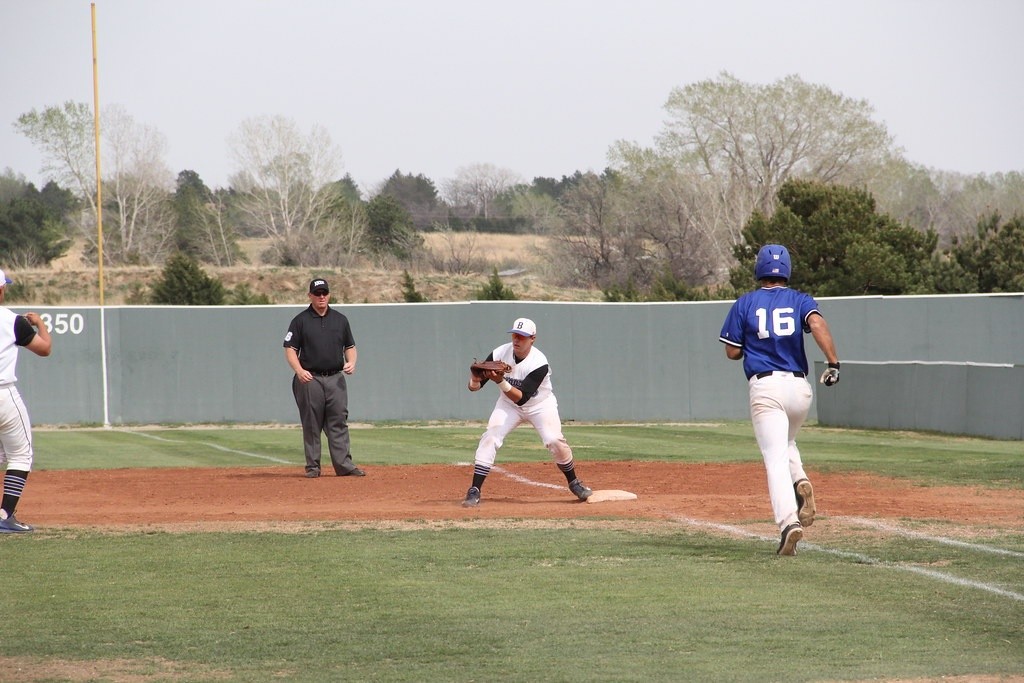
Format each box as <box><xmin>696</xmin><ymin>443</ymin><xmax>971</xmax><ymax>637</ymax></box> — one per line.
<box><xmin>754</xmin><ymin>245</ymin><xmax>791</xmax><ymax>283</ymax></box>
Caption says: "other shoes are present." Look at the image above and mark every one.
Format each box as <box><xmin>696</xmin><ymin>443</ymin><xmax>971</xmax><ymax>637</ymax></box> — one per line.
<box><xmin>346</xmin><ymin>469</ymin><xmax>365</xmax><ymax>476</ymax></box>
<box><xmin>304</xmin><ymin>470</ymin><xmax>320</xmax><ymax>478</ymax></box>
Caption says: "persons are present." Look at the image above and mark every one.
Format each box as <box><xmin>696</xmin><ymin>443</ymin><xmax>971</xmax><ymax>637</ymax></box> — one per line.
<box><xmin>283</xmin><ymin>279</ymin><xmax>366</xmax><ymax>478</ymax></box>
<box><xmin>0</xmin><ymin>268</ymin><xmax>52</xmax><ymax>533</ymax></box>
<box><xmin>719</xmin><ymin>245</ymin><xmax>841</xmax><ymax>556</ymax></box>
<box><xmin>461</xmin><ymin>317</ymin><xmax>592</xmax><ymax>508</ymax></box>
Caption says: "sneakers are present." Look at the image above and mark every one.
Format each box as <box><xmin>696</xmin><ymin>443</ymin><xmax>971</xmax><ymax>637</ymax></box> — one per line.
<box><xmin>567</xmin><ymin>477</ymin><xmax>592</xmax><ymax>502</ymax></box>
<box><xmin>793</xmin><ymin>478</ymin><xmax>816</xmax><ymax>527</ymax></box>
<box><xmin>777</xmin><ymin>522</ymin><xmax>804</xmax><ymax>556</ymax></box>
<box><xmin>0</xmin><ymin>510</ymin><xmax>34</xmax><ymax>533</ymax></box>
<box><xmin>462</xmin><ymin>487</ymin><xmax>481</xmax><ymax>507</ymax></box>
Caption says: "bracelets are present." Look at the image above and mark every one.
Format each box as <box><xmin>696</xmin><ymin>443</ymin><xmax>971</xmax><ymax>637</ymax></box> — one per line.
<box><xmin>497</xmin><ymin>379</ymin><xmax>512</xmax><ymax>392</ymax></box>
<box><xmin>470</xmin><ymin>378</ymin><xmax>480</xmax><ymax>388</ymax></box>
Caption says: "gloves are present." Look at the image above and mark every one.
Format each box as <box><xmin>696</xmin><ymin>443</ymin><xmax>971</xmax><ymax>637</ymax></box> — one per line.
<box><xmin>819</xmin><ymin>362</ymin><xmax>840</xmax><ymax>386</ymax></box>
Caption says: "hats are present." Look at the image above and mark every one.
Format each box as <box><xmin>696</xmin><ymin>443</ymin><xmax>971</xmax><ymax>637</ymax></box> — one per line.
<box><xmin>310</xmin><ymin>278</ymin><xmax>329</xmax><ymax>294</ymax></box>
<box><xmin>0</xmin><ymin>269</ymin><xmax>13</xmax><ymax>286</ymax></box>
<box><xmin>506</xmin><ymin>317</ymin><xmax>537</xmax><ymax>337</ymax></box>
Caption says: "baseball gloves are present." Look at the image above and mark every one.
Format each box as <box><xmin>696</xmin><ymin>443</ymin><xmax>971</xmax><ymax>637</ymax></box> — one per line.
<box><xmin>470</xmin><ymin>361</ymin><xmax>512</xmax><ymax>380</ymax></box>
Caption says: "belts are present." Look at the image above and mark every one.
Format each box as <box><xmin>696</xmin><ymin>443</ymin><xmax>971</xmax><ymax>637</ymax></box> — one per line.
<box><xmin>756</xmin><ymin>370</ymin><xmax>805</xmax><ymax>379</ymax></box>
<box><xmin>309</xmin><ymin>370</ymin><xmax>340</xmax><ymax>376</ymax></box>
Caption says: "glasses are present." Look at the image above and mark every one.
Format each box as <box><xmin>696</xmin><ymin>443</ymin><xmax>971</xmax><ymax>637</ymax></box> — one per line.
<box><xmin>313</xmin><ymin>290</ymin><xmax>328</xmax><ymax>297</ymax></box>
<box><xmin>511</xmin><ymin>332</ymin><xmax>528</xmax><ymax>340</ymax></box>
